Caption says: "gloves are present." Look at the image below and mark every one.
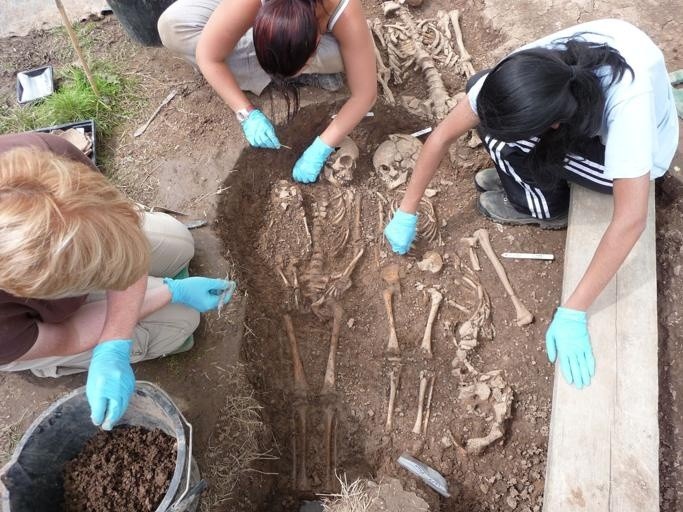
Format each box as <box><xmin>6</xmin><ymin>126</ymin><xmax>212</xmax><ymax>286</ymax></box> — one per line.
<box><xmin>86</xmin><ymin>339</ymin><xmax>135</xmax><ymax>431</ymax></box>
<box><xmin>384</xmin><ymin>208</ymin><xmax>417</xmax><ymax>255</ymax></box>
<box><xmin>163</xmin><ymin>277</ymin><xmax>236</xmax><ymax>313</ymax></box>
<box><xmin>241</xmin><ymin>109</ymin><xmax>281</xmax><ymax>149</ymax></box>
<box><xmin>292</xmin><ymin>135</ymin><xmax>335</xmax><ymax>183</ymax></box>
<box><xmin>545</xmin><ymin>306</ymin><xmax>595</xmax><ymax>388</ymax></box>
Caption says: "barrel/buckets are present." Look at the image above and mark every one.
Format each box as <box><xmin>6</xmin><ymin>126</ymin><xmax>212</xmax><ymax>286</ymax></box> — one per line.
<box><xmin>0</xmin><ymin>380</ymin><xmax>201</xmax><ymax>512</ymax></box>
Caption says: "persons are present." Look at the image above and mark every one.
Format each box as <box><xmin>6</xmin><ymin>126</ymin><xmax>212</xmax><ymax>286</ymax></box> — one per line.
<box><xmin>0</xmin><ymin>133</ymin><xmax>236</xmax><ymax>432</ymax></box>
<box><xmin>384</xmin><ymin>18</ymin><xmax>680</xmax><ymax>387</ymax></box>
<box><xmin>157</xmin><ymin>1</ymin><xmax>378</xmax><ymax>184</ymax></box>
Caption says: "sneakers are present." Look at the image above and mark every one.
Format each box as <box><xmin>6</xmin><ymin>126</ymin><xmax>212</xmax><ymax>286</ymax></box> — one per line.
<box><xmin>478</xmin><ymin>189</ymin><xmax>568</xmax><ymax>230</ymax></box>
<box><xmin>288</xmin><ymin>73</ymin><xmax>344</xmax><ymax>92</ymax></box>
<box><xmin>474</xmin><ymin>168</ymin><xmax>504</xmax><ymax>192</ymax></box>
